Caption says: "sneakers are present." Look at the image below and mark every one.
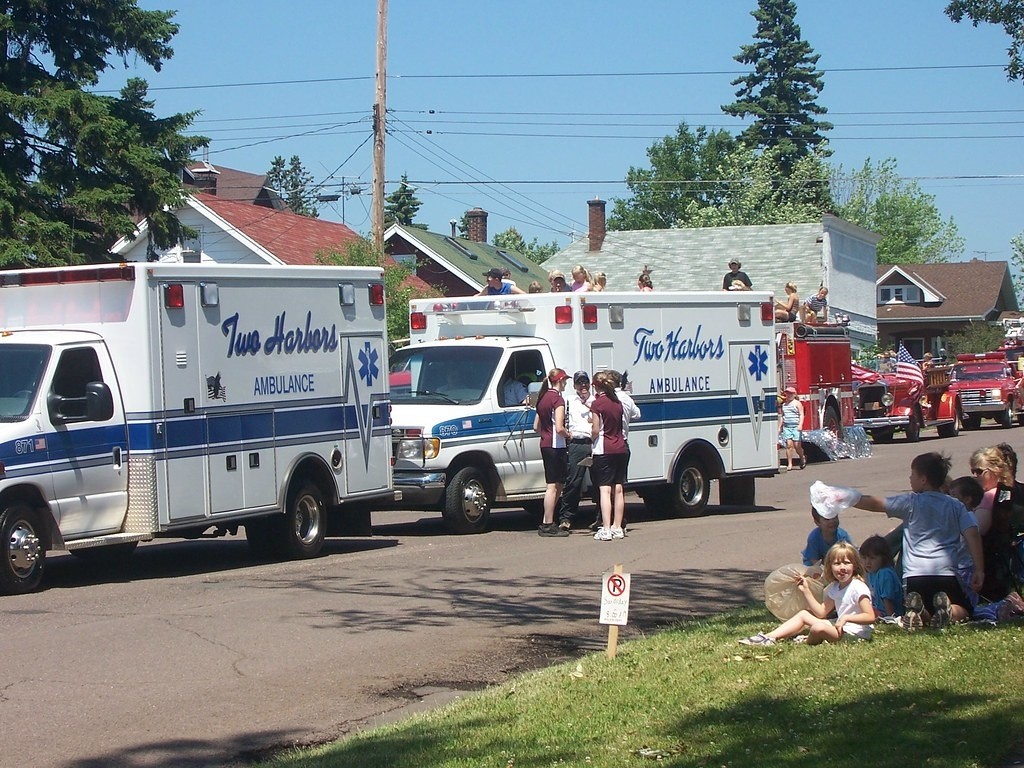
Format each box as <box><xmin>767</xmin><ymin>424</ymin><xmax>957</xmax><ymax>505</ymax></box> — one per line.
<box><xmin>794</xmin><ymin>635</ymin><xmax>808</xmax><ymax>645</ymax></box>
<box><xmin>737</xmin><ymin>630</ymin><xmax>776</xmax><ymax>647</ymax></box>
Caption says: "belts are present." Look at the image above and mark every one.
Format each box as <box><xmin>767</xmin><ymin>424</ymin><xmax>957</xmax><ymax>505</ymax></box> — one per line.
<box><xmin>570</xmin><ymin>438</ymin><xmax>592</xmax><ymax>445</ymax></box>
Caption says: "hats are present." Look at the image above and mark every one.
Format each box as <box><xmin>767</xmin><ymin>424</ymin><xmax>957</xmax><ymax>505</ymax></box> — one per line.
<box><xmin>784</xmin><ymin>387</ymin><xmax>796</xmax><ymax>394</ymax></box>
<box><xmin>482</xmin><ymin>267</ymin><xmax>501</xmax><ymax>279</ymax></box>
<box><xmin>574</xmin><ymin>370</ymin><xmax>589</xmax><ymax>382</ymax></box>
<box><xmin>643</xmin><ymin>264</ymin><xmax>654</xmax><ymax>272</ymax></box>
<box><xmin>728</xmin><ymin>257</ymin><xmax>740</xmax><ymax>265</ymax></box>
<box><xmin>550</xmin><ymin>368</ymin><xmax>572</xmax><ymax>381</ymax></box>
<box><xmin>547</xmin><ymin>269</ymin><xmax>566</xmax><ymax>285</ymax></box>
<box><xmin>499</xmin><ymin>266</ymin><xmax>512</xmax><ymax>277</ymax></box>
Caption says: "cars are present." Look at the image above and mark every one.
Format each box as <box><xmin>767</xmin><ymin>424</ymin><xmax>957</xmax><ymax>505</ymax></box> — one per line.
<box><xmin>853</xmin><ymin>316</ymin><xmax>1024</xmax><ymax>443</ymax></box>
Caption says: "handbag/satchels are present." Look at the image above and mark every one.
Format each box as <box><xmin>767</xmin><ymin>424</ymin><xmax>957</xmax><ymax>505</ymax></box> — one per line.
<box><xmin>764</xmin><ymin>563</ymin><xmax>827</xmax><ymax>623</ymax></box>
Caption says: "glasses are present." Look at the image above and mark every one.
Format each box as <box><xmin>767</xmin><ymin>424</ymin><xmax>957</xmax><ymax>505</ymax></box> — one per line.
<box><xmin>971</xmin><ymin>468</ymin><xmax>985</xmax><ymax>476</ymax></box>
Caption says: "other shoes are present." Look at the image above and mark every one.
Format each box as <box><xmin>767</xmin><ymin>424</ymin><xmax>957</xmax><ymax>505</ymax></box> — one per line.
<box><xmin>559</xmin><ymin>522</ymin><xmax>570</xmax><ymax>530</ymax></box>
<box><xmin>538</xmin><ymin>523</ymin><xmax>569</xmax><ymax>537</ymax></box>
<box><xmin>904</xmin><ymin>592</ymin><xmax>923</xmax><ymax>633</ymax></box>
<box><xmin>594</xmin><ymin>526</ymin><xmax>612</xmax><ymax>541</ymax></box>
<box><xmin>590</xmin><ymin>521</ymin><xmax>603</xmax><ymax>531</ymax></box>
<box><xmin>787</xmin><ymin>467</ymin><xmax>792</xmax><ymax>470</ymax></box>
<box><xmin>610</xmin><ymin>526</ymin><xmax>624</xmax><ymax>539</ymax></box>
<box><xmin>800</xmin><ymin>456</ymin><xmax>807</xmax><ymax>469</ymax></box>
<box><xmin>930</xmin><ymin>591</ymin><xmax>953</xmax><ymax>634</ymax></box>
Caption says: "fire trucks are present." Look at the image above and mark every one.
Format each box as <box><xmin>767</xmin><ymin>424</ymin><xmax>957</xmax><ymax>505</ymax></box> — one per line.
<box><xmin>386</xmin><ymin>338</ymin><xmax>412</xmax><ymax>400</ymax></box>
<box><xmin>775</xmin><ymin>313</ymin><xmax>855</xmax><ymax>467</ymax></box>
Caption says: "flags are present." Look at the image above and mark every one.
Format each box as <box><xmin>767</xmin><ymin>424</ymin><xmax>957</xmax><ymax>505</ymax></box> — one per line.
<box><xmin>851</xmin><ymin>364</ymin><xmax>882</xmax><ymax>383</ymax></box>
<box><xmin>896</xmin><ymin>345</ymin><xmax>924</xmax><ymax>401</ymax></box>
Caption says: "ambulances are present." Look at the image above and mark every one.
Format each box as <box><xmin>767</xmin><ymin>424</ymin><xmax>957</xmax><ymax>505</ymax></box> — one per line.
<box><xmin>0</xmin><ymin>260</ymin><xmax>402</xmax><ymax>596</ymax></box>
<box><xmin>388</xmin><ymin>291</ymin><xmax>787</xmax><ymax>535</ymax></box>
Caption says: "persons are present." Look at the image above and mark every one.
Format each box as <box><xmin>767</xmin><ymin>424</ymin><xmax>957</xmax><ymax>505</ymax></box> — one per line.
<box><xmin>778</xmin><ymin>387</ymin><xmax>806</xmax><ymax>471</ymax></box>
<box><xmin>774</xmin><ymin>282</ymin><xmax>799</xmax><ymax>322</ymax></box>
<box><xmin>799</xmin><ymin>288</ymin><xmax>828</xmax><ymax>323</ymax></box>
<box><xmin>802</xmin><ymin>507</ymin><xmax>853</xmax><ymax>568</ymax></box>
<box><xmin>723</xmin><ymin>258</ymin><xmax>753</xmax><ymax>291</ymax></box>
<box><xmin>502</xmin><ymin>370</ymin><xmax>528</xmax><ymax>405</ymax></box>
<box><xmin>638</xmin><ymin>274</ymin><xmax>652</xmax><ymax>292</ymax></box>
<box><xmin>738</xmin><ymin>543</ymin><xmax>875</xmax><ymax>645</ymax></box>
<box><xmin>532</xmin><ymin>369</ymin><xmax>641</xmax><ymax>540</ymax></box>
<box><xmin>548</xmin><ymin>266</ymin><xmax>606</xmax><ymax>292</ymax></box>
<box><xmin>922</xmin><ymin>352</ymin><xmax>933</xmax><ymax>370</ymax></box>
<box><xmin>884</xmin><ymin>354</ymin><xmax>896</xmax><ymax>372</ymax></box>
<box><xmin>436</xmin><ymin>367</ymin><xmax>469</xmax><ymax>390</ymax></box>
<box><xmin>475</xmin><ymin>267</ymin><xmax>525</xmax><ymax>296</ymax></box>
<box><xmin>858</xmin><ymin>536</ymin><xmax>904</xmax><ymax>618</ymax></box>
<box><xmin>810</xmin><ymin>443</ymin><xmax>1024</xmax><ymax>628</ymax></box>
